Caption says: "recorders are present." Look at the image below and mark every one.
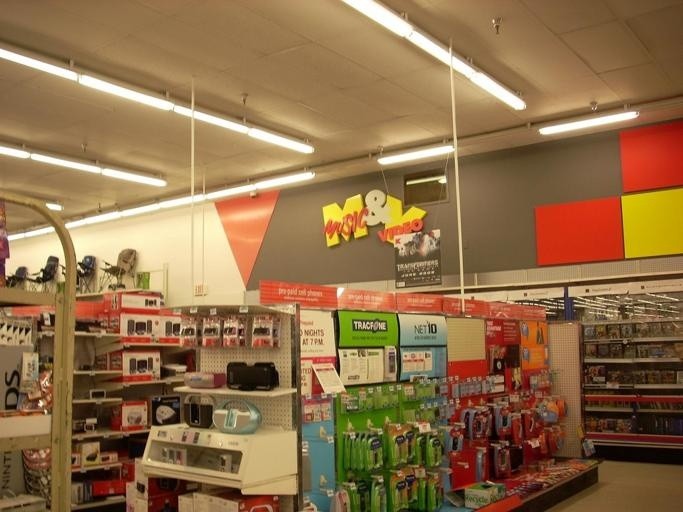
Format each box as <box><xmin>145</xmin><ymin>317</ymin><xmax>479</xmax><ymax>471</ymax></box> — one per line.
<box><xmin>184</xmin><ymin>393</ymin><xmax>215</xmax><ymax>428</ymax></box>
<box><xmin>213</xmin><ymin>398</ymin><xmax>262</xmax><ymax>434</ymax></box>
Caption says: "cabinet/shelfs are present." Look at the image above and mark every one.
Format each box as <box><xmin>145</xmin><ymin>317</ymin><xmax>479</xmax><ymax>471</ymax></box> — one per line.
<box><xmin>579</xmin><ymin>279</ymin><xmax>683</xmax><ymax>460</ymax></box>
<box><xmin>141</xmin><ymin>301</ymin><xmax>303</xmax><ymax>512</ymax></box>
<box><xmin>71</xmin><ymin>330</ymin><xmax>196</xmax><ymax>512</ymax></box>
<box><xmin>0</xmin><ymin>191</ymin><xmax>77</xmax><ymax>511</ymax></box>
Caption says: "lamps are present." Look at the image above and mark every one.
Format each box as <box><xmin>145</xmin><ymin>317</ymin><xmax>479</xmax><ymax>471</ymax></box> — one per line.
<box><xmin>377</xmin><ymin>144</ymin><xmax>456</xmax><ymax>166</ymax></box>
<box><xmin>538</xmin><ymin>104</ymin><xmax>640</xmax><ymax>136</ymax></box>
<box><xmin>1</xmin><ymin>170</ymin><xmax>316</xmax><ymax>240</ymax></box>
<box><xmin>342</xmin><ymin>1</ymin><xmax>527</xmax><ymax>112</ymax></box>
<box><xmin>1</xmin><ymin>143</ymin><xmax>169</xmax><ymax>188</ymax></box>
<box><xmin>1</xmin><ymin>45</ymin><xmax>316</xmax><ymax>156</ymax></box>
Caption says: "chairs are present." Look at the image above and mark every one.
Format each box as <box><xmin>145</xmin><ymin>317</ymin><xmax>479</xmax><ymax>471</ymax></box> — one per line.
<box><xmin>6</xmin><ymin>248</ymin><xmax>136</xmax><ymax>295</ymax></box>
